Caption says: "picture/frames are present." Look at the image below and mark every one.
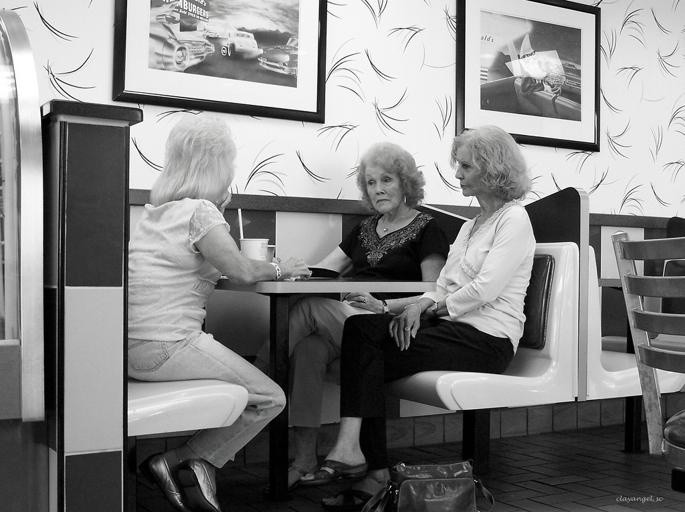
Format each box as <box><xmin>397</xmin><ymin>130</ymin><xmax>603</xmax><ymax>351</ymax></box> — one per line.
<box><xmin>112</xmin><ymin>0</ymin><xmax>329</xmax><ymax>122</ymax></box>
<box><xmin>455</xmin><ymin>0</ymin><xmax>603</xmax><ymax>152</ymax></box>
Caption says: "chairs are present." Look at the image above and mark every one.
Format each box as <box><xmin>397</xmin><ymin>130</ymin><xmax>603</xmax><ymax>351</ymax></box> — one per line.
<box><xmin>611</xmin><ymin>230</ymin><xmax>685</xmax><ymax>470</ymax></box>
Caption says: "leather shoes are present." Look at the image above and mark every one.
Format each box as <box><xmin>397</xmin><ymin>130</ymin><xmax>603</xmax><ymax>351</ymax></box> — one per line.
<box><xmin>275</xmin><ymin>458</ymin><xmax>319</xmax><ymax>489</ymax></box>
<box><xmin>136</xmin><ymin>451</ymin><xmax>188</xmax><ymax>510</ymax></box>
<box><xmin>174</xmin><ymin>458</ymin><xmax>222</xmax><ymax>511</ymax></box>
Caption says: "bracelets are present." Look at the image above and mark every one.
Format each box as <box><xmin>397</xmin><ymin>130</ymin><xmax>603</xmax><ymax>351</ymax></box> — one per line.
<box><xmin>271</xmin><ymin>261</ymin><xmax>283</xmax><ymax>280</ymax></box>
<box><xmin>382</xmin><ymin>298</ymin><xmax>389</xmax><ymax>314</ymax></box>
<box><xmin>432</xmin><ymin>301</ymin><xmax>439</xmax><ymax>317</ymax></box>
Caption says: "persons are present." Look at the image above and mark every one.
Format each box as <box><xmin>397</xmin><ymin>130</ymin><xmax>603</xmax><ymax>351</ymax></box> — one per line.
<box><xmin>128</xmin><ymin>114</ymin><xmax>287</xmax><ymax>512</ymax></box>
<box><xmin>252</xmin><ymin>141</ymin><xmax>450</xmax><ymax>487</ymax></box>
<box><xmin>296</xmin><ymin>123</ymin><xmax>538</xmax><ymax>508</ymax></box>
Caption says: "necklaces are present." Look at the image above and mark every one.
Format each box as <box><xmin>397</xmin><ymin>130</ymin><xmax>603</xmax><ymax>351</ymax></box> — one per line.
<box><xmin>382</xmin><ymin>219</ymin><xmax>395</xmax><ymax>231</ymax></box>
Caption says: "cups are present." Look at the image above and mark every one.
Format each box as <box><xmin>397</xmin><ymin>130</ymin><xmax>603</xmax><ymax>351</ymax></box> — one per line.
<box><xmin>268</xmin><ymin>244</ymin><xmax>276</xmax><ymax>262</ymax></box>
<box><xmin>238</xmin><ymin>238</ymin><xmax>270</xmax><ymax>262</ymax></box>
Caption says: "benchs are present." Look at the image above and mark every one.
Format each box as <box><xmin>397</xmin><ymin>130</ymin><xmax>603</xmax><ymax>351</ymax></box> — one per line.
<box><xmin>324</xmin><ymin>241</ymin><xmax>580</xmax><ymax>470</ymax></box>
<box><xmin>126</xmin><ymin>372</ymin><xmax>250</xmax><ymax>511</ymax></box>
<box><xmin>589</xmin><ymin>245</ymin><xmax>685</xmax><ymax>454</ymax></box>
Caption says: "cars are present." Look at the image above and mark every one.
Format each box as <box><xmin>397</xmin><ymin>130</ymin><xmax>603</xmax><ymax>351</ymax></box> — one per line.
<box><xmin>150</xmin><ymin>0</ymin><xmax>217</xmax><ymax>73</ymax></box>
<box><xmin>555</xmin><ymin>60</ymin><xmax>581</xmax><ymax>98</ymax></box>
<box><xmin>256</xmin><ymin>35</ymin><xmax>298</xmax><ymax>80</ymax></box>
<box><xmin>217</xmin><ymin>30</ymin><xmax>263</xmax><ymax>63</ymax></box>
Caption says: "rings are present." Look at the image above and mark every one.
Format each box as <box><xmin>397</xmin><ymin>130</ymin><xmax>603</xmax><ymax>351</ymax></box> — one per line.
<box><xmin>404</xmin><ymin>327</ymin><xmax>410</xmax><ymax>331</ymax></box>
<box><xmin>362</xmin><ymin>298</ymin><xmax>366</xmax><ymax>303</ymax></box>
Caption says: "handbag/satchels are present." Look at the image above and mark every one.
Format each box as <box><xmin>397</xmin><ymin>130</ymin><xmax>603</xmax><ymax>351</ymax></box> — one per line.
<box><xmin>360</xmin><ymin>457</ymin><xmax>495</xmax><ymax>512</ymax></box>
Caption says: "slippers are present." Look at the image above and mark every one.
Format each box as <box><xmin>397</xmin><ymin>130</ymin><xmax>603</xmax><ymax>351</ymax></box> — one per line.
<box><xmin>321</xmin><ymin>484</ymin><xmax>379</xmax><ymax>512</ymax></box>
<box><xmin>298</xmin><ymin>457</ymin><xmax>369</xmax><ymax>487</ymax></box>
